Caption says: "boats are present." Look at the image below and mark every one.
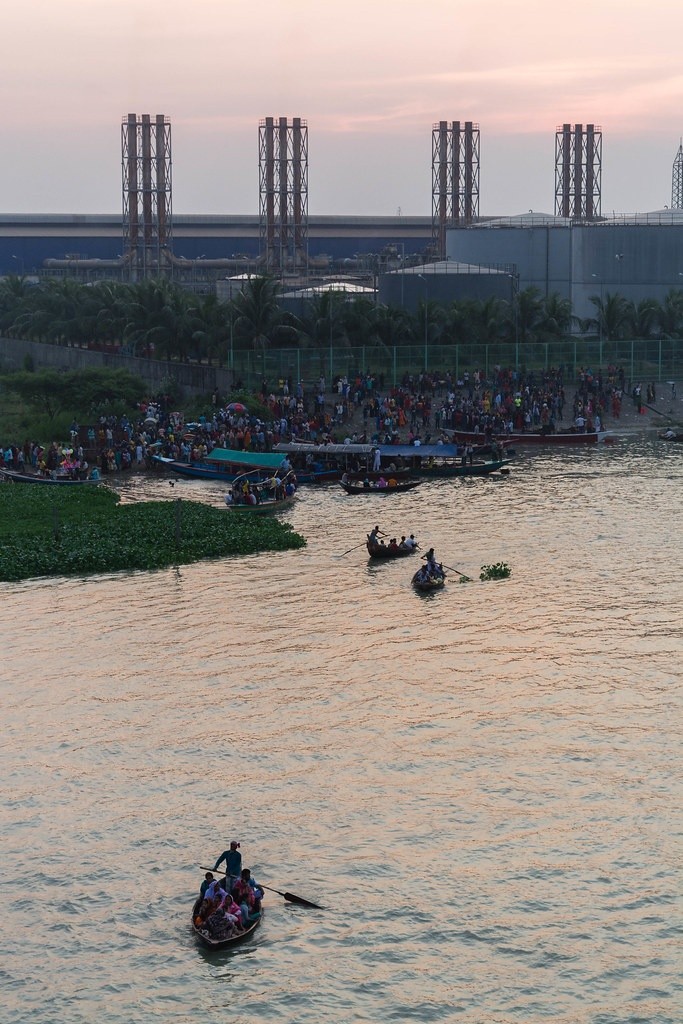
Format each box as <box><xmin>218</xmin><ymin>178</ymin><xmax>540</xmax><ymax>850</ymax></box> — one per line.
<box><xmin>659</xmin><ymin>433</ymin><xmax>682</xmax><ymax>442</ymax></box>
<box><xmin>413</xmin><ymin>564</ymin><xmax>445</xmax><ymax>588</ymax></box>
<box><xmin>439</xmin><ymin>427</ymin><xmax>617</xmax><ymax>445</ymax></box>
<box><xmin>339</xmin><ymin>479</ymin><xmax>421</xmax><ymax>492</ymax></box>
<box><xmin>192</xmin><ymin>876</ymin><xmax>261</xmax><ymax>947</ymax></box>
<box><xmin>0</xmin><ymin>469</ymin><xmax>99</xmax><ymax>486</ymax></box>
<box><xmin>151</xmin><ymin>448</ymin><xmax>342</xmax><ymax>483</ymax></box>
<box><xmin>275</xmin><ymin>440</ymin><xmax>510</xmax><ymax>476</ymax></box>
<box><xmin>226</xmin><ymin>482</ymin><xmax>295</xmax><ymax>515</ymax></box>
<box><xmin>366</xmin><ymin>541</ymin><xmax>416</xmax><ymax>556</ymax></box>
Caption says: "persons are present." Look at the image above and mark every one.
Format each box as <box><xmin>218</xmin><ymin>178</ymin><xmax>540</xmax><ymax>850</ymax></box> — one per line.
<box><xmin>280</xmin><ymin>455</ymin><xmax>290</xmax><ymax>470</ymax></box>
<box><xmin>231</xmin><ymin>378</ymin><xmax>243</xmax><ymax>392</ymax></box>
<box><xmin>0</xmin><ymin>440</ymin><xmax>100</xmax><ymax>480</ymax></box>
<box><xmin>196</xmin><ymin>841</ymin><xmax>265</xmax><ymax>939</ymax></box>
<box><xmin>149</xmin><ymin>341</ymin><xmax>155</xmax><ymax>348</ymax></box>
<box><xmin>69</xmin><ymin>401</ymin><xmax>286</xmax><ymax>472</ymax></box>
<box><xmin>225</xmin><ymin>467</ymin><xmax>297</xmax><ymax>505</ymax></box>
<box><xmin>671</xmin><ymin>384</ymin><xmax>676</xmax><ymax>399</ymax></box>
<box><xmin>214</xmin><ymin>387</ymin><xmax>218</xmax><ymax>395</ymax></box>
<box><xmin>664</xmin><ymin>428</ymin><xmax>674</xmax><ymax>437</ymax></box>
<box><xmin>415</xmin><ymin>548</ymin><xmax>446</xmax><ymax>583</ymax></box>
<box><xmin>211</xmin><ymin>393</ymin><xmax>217</xmax><ymax>410</ymax></box>
<box><xmin>259</xmin><ymin>362</ymin><xmax>656</xmax><ymax>471</ymax></box>
<box><xmin>370</xmin><ymin>526</ymin><xmax>422</xmax><ymax>550</ymax></box>
<box><xmin>341</xmin><ymin>470</ymin><xmax>398</xmax><ymax>486</ymax></box>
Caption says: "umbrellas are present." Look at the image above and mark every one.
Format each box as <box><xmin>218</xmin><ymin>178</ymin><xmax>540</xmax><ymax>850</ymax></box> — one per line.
<box><xmin>225</xmin><ymin>403</ymin><xmax>247</xmax><ymax>415</ymax></box>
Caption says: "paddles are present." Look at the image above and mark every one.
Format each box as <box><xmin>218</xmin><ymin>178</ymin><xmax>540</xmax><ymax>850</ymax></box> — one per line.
<box><xmin>199</xmin><ymin>866</ymin><xmax>324</xmax><ymax>909</ymax></box>
<box><xmin>340</xmin><ymin>534</ymin><xmax>391</xmax><ymax>556</ymax></box>
<box><xmin>421</xmin><ymin>557</ymin><xmax>465</xmax><ymax>578</ymax></box>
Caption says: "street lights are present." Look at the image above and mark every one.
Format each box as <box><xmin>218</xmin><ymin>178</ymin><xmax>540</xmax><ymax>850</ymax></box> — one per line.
<box><xmin>419</xmin><ymin>273</ymin><xmax>428</xmax><ymax>372</ymax></box>
<box><xmin>508</xmin><ymin>274</ymin><xmax>518</xmax><ymax>371</ymax></box>
<box><xmin>592</xmin><ymin>274</ymin><xmax>602</xmax><ymax>370</ymax></box>
